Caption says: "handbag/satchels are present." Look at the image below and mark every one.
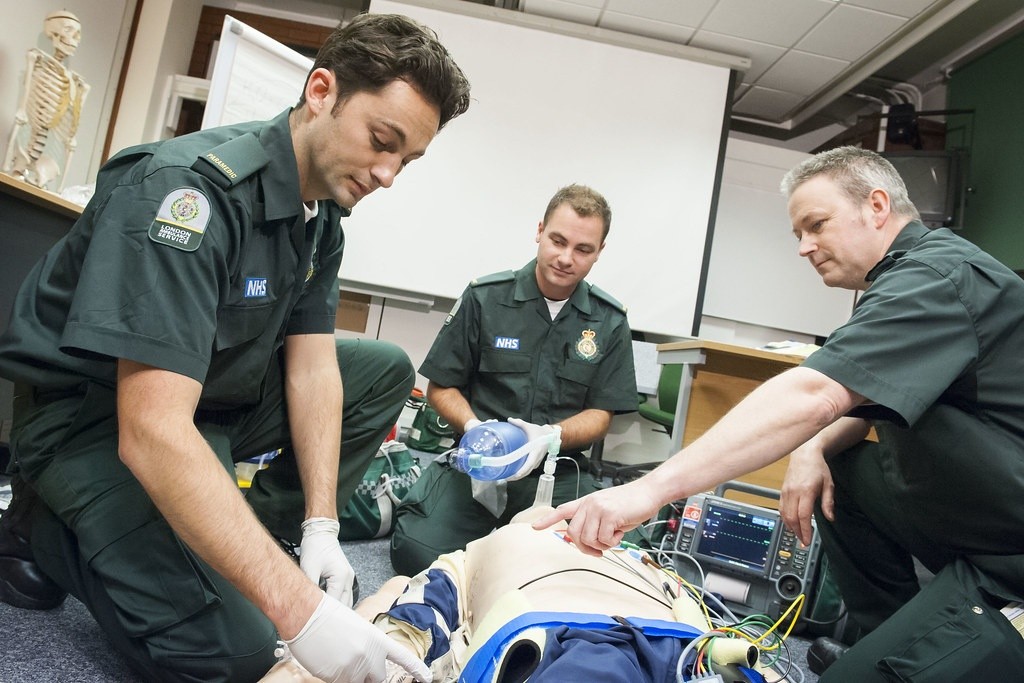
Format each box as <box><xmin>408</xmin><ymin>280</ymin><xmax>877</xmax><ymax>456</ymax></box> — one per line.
<box><xmin>407</xmin><ymin>402</ymin><xmax>459</xmax><ymax>454</ymax></box>
<box><xmin>336</xmin><ymin>440</ymin><xmax>428</xmax><ymax>541</ymax></box>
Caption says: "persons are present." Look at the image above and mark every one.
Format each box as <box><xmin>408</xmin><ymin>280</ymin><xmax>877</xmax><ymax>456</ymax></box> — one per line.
<box><xmin>390</xmin><ymin>182</ymin><xmax>658</xmax><ymax>578</ymax></box>
<box><xmin>365</xmin><ymin>506</ymin><xmax>788</xmax><ymax>683</ymax></box>
<box><xmin>0</xmin><ymin>14</ymin><xmax>471</xmax><ymax>683</ymax></box>
<box><xmin>532</xmin><ymin>145</ymin><xmax>1024</xmax><ymax>683</ymax></box>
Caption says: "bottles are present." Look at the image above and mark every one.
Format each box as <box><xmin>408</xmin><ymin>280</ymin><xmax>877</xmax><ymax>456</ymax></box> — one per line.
<box><xmin>508</xmin><ymin>439</ymin><xmax>569</xmax><ymax>533</ymax></box>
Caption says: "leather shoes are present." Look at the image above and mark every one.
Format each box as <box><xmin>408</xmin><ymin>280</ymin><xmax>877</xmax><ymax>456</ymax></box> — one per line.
<box><xmin>0</xmin><ymin>497</ymin><xmax>68</xmax><ymax>611</ymax></box>
<box><xmin>808</xmin><ymin>636</ymin><xmax>852</xmax><ymax>674</ymax></box>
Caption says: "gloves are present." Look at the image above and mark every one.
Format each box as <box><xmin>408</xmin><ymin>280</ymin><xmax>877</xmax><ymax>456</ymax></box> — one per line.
<box><xmin>464</xmin><ymin>418</ymin><xmax>498</xmax><ymax>456</ymax></box>
<box><xmin>300</xmin><ymin>517</ymin><xmax>355</xmax><ymax>608</ymax></box>
<box><xmin>507</xmin><ymin>417</ymin><xmax>554</xmax><ymax>480</ymax></box>
<box><xmin>279</xmin><ymin>590</ymin><xmax>432</xmax><ymax>683</ymax></box>
